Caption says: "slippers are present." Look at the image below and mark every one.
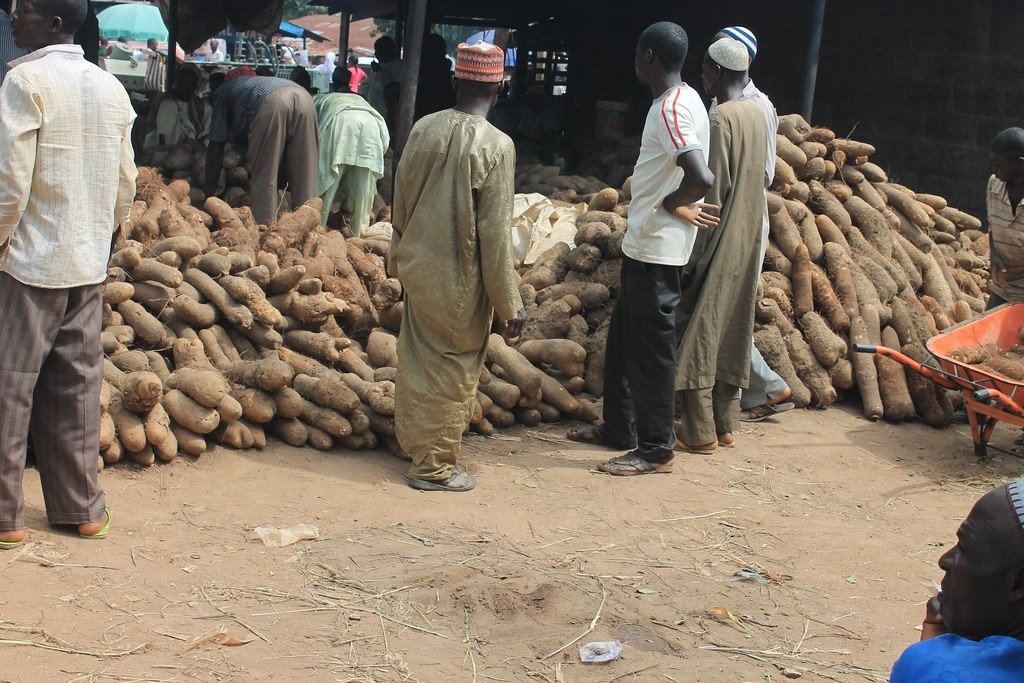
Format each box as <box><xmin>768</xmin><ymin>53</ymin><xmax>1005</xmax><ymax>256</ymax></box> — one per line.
<box><xmin>0</xmin><ymin>541</ymin><xmax>22</xmax><ymax>549</ymax></box>
<box><xmin>409</xmin><ymin>471</ymin><xmax>477</xmax><ymax>492</ymax></box>
<box><xmin>597</xmin><ymin>451</ymin><xmax>673</xmax><ymax>475</ymax></box>
<box><xmin>79</xmin><ymin>504</ymin><xmax>111</xmax><ymax>539</ymax></box>
<box><xmin>567</xmin><ymin>424</ymin><xmax>636</xmax><ymax>450</ymax></box>
<box><xmin>675</xmin><ymin>427</ymin><xmax>736</xmax><ymax>454</ymax></box>
<box><xmin>740</xmin><ymin>402</ymin><xmax>795</xmax><ymax>422</ymax></box>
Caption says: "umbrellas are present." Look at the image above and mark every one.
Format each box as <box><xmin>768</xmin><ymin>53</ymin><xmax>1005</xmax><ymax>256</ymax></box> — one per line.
<box><xmin>94</xmin><ymin>3</ymin><xmax>170</xmax><ymax>43</ymax></box>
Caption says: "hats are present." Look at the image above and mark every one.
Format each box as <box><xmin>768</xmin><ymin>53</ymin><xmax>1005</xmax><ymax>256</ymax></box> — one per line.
<box><xmin>708</xmin><ymin>38</ymin><xmax>749</xmax><ymax>72</ymax></box>
<box><xmin>719</xmin><ymin>25</ymin><xmax>758</xmax><ymax>61</ymax></box>
<box><xmin>455</xmin><ymin>39</ymin><xmax>504</xmax><ymax>83</ymax></box>
<box><xmin>224</xmin><ymin>66</ymin><xmax>256</xmax><ymax>82</ymax></box>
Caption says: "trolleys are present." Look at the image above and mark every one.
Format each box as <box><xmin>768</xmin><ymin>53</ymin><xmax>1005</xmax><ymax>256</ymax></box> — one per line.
<box><xmin>852</xmin><ymin>300</ymin><xmax>1024</xmax><ymax>459</ymax></box>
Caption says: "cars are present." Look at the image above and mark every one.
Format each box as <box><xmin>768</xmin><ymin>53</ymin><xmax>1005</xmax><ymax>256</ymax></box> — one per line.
<box><xmin>99</xmin><ymin>42</ymin><xmax>183</xmax><ymax>62</ymax></box>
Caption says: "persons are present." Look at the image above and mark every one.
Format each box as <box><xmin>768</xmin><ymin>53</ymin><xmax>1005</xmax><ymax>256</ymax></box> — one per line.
<box><xmin>890</xmin><ymin>480</ymin><xmax>1024</xmax><ymax>683</ymax></box>
<box><xmin>0</xmin><ymin>0</ymin><xmax>140</xmax><ymax>550</ymax></box>
<box><xmin>674</xmin><ymin>26</ymin><xmax>795</xmax><ymax>454</ymax></box>
<box><xmin>565</xmin><ymin>21</ymin><xmax>718</xmax><ymax>476</ymax></box>
<box><xmin>953</xmin><ymin>127</ymin><xmax>1024</xmax><ymax>445</ymax></box>
<box><xmin>97</xmin><ymin>36</ymin><xmax>457</xmax><ymax>237</ymax></box>
<box><xmin>386</xmin><ymin>42</ymin><xmax>530</xmax><ymax>490</ymax></box>
